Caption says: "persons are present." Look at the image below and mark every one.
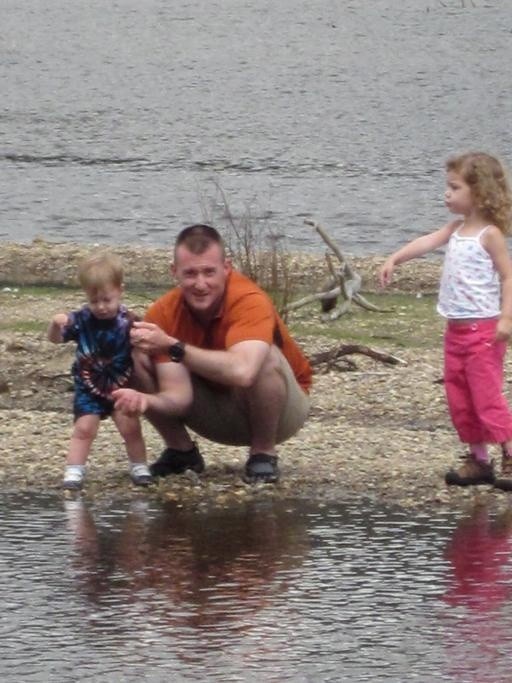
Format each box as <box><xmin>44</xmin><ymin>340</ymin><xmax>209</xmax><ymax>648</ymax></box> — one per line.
<box><xmin>378</xmin><ymin>150</ymin><xmax>512</xmax><ymax>489</ymax></box>
<box><xmin>109</xmin><ymin>224</ymin><xmax>310</xmax><ymax>484</ymax></box>
<box><xmin>46</xmin><ymin>257</ymin><xmax>155</xmax><ymax>487</ymax></box>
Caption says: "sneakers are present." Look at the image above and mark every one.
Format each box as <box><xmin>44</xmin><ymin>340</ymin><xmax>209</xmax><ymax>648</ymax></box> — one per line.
<box><xmin>496</xmin><ymin>455</ymin><xmax>512</xmax><ymax>489</ymax></box>
<box><xmin>63</xmin><ymin>469</ymin><xmax>85</xmax><ymax>489</ymax></box>
<box><xmin>243</xmin><ymin>450</ymin><xmax>281</xmax><ymax>484</ymax></box>
<box><xmin>148</xmin><ymin>440</ymin><xmax>205</xmax><ymax>476</ymax></box>
<box><xmin>445</xmin><ymin>453</ymin><xmax>496</xmax><ymax>486</ymax></box>
<box><xmin>130</xmin><ymin>465</ymin><xmax>154</xmax><ymax>485</ymax></box>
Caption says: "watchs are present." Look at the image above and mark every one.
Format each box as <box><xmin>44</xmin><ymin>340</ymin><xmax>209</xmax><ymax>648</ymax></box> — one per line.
<box><xmin>167</xmin><ymin>340</ymin><xmax>187</xmax><ymax>363</ymax></box>
